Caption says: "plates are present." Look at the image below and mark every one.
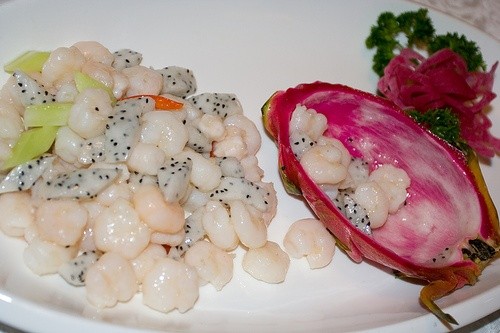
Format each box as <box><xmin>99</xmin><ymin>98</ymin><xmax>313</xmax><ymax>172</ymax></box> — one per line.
<box><xmin>0</xmin><ymin>0</ymin><xmax>500</xmax><ymax>332</ymax></box>
